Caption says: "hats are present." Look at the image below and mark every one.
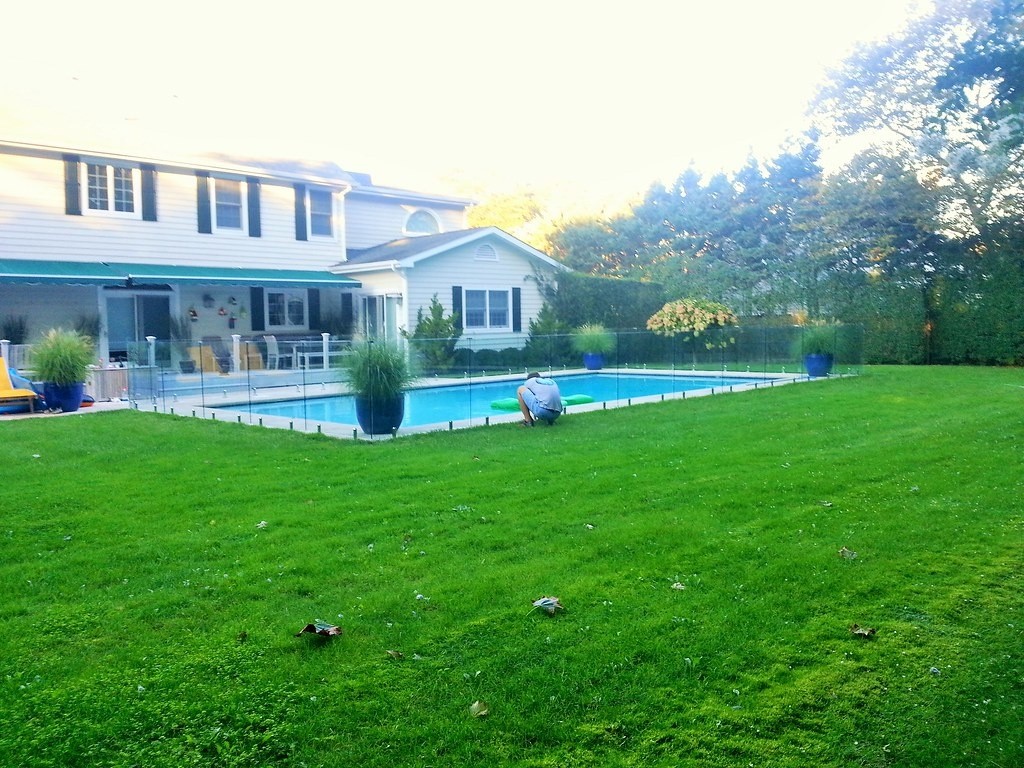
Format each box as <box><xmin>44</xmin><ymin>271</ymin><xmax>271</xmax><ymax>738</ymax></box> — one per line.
<box><xmin>527</xmin><ymin>372</ymin><xmax>540</xmax><ymax>379</ymax></box>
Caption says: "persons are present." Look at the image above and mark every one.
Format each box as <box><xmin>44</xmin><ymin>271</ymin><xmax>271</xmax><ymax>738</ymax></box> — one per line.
<box><xmin>515</xmin><ymin>371</ymin><xmax>563</xmax><ymax>428</ymax></box>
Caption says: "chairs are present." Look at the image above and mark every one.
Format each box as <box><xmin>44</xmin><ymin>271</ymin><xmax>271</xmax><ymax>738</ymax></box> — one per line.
<box><xmin>265</xmin><ymin>335</ymin><xmax>295</xmax><ymax>371</ymax></box>
<box><xmin>0</xmin><ymin>357</ymin><xmax>37</xmax><ymax>415</ymax></box>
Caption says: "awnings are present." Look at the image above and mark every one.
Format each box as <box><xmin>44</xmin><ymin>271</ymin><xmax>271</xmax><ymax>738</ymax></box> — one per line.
<box><xmin>1</xmin><ymin>257</ymin><xmax>364</xmax><ymax>289</ymax></box>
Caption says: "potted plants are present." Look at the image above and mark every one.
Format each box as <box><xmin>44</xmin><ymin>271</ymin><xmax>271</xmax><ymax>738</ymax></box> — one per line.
<box><xmin>342</xmin><ymin>335</ymin><xmax>409</xmax><ymax>434</ymax></box>
<box><xmin>167</xmin><ymin>316</ymin><xmax>196</xmax><ymax>374</ymax></box>
<box><xmin>33</xmin><ymin>326</ymin><xmax>95</xmax><ymax>412</ymax></box>
<box><xmin>791</xmin><ymin>324</ymin><xmax>840</xmax><ymax>378</ymax></box>
<box><xmin>569</xmin><ymin>323</ymin><xmax>618</xmax><ymax>370</ymax></box>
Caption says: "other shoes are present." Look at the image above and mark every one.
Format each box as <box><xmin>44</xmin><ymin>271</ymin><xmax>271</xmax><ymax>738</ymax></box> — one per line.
<box><xmin>523</xmin><ymin>420</ymin><xmax>532</xmax><ymax>427</ymax></box>
